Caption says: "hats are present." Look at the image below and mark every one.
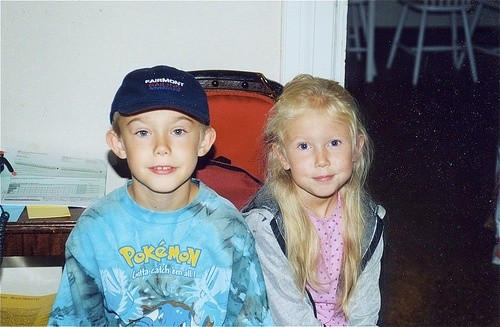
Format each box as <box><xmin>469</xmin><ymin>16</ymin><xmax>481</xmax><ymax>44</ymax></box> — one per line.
<box><xmin>109</xmin><ymin>64</ymin><xmax>210</xmax><ymax>125</ymax></box>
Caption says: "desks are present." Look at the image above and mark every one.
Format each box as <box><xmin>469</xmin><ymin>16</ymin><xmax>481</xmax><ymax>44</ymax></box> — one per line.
<box><xmin>0</xmin><ymin>205</ymin><xmax>84</xmax><ymax>257</ymax></box>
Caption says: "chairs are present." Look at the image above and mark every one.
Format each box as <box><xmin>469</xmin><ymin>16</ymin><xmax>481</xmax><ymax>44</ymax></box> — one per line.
<box><xmin>384</xmin><ymin>0</ymin><xmax>479</xmax><ymax>84</ymax></box>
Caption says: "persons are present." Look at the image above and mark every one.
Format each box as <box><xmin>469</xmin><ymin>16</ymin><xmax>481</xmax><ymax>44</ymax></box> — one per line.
<box><xmin>46</xmin><ymin>65</ymin><xmax>275</xmax><ymax>327</ymax></box>
<box><xmin>238</xmin><ymin>73</ymin><xmax>386</xmax><ymax>327</ymax></box>
